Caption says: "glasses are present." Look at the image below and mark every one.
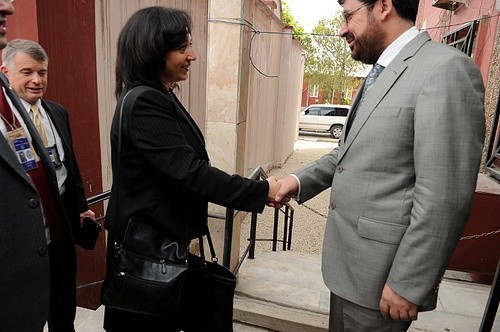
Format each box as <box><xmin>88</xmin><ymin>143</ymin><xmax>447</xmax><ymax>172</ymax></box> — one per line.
<box><xmin>342</xmin><ymin>1</ymin><xmax>376</xmax><ymax>23</ymax></box>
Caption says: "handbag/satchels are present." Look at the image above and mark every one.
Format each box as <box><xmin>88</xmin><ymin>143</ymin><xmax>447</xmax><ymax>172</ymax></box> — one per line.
<box><xmin>102</xmin><ymin>240</ymin><xmax>188</xmax><ymax>319</ymax></box>
<box><xmin>184</xmin><ymin>250</ymin><xmax>237</xmax><ymax>332</ymax></box>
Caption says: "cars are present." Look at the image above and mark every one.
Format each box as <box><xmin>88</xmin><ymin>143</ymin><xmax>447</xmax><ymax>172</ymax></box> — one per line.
<box><xmin>300</xmin><ymin>103</ymin><xmax>351</xmax><ymax>139</ymax></box>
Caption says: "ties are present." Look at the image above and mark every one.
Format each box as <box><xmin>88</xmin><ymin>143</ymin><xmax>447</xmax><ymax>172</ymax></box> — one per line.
<box><xmin>346</xmin><ymin>63</ymin><xmax>383</xmax><ymax>139</ymax></box>
<box><xmin>0</xmin><ymin>86</ymin><xmax>67</xmax><ymax>241</ymax></box>
<box><xmin>31</xmin><ymin>105</ymin><xmax>49</xmax><ymax>148</ymax></box>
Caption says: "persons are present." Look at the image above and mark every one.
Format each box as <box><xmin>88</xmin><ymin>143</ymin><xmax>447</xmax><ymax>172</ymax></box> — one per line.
<box><xmin>101</xmin><ymin>6</ymin><xmax>295</xmax><ymax>332</ymax></box>
<box><xmin>20</xmin><ymin>152</ymin><xmax>27</xmax><ymax>162</ymax></box>
<box><xmin>0</xmin><ymin>39</ymin><xmax>96</xmax><ymax>332</ymax></box>
<box><xmin>266</xmin><ymin>0</ymin><xmax>486</xmax><ymax>332</ymax></box>
<box><xmin>0</xmin><ymin>0</ymin><xmax>77</xmax><ymax>332</ymax></box>
